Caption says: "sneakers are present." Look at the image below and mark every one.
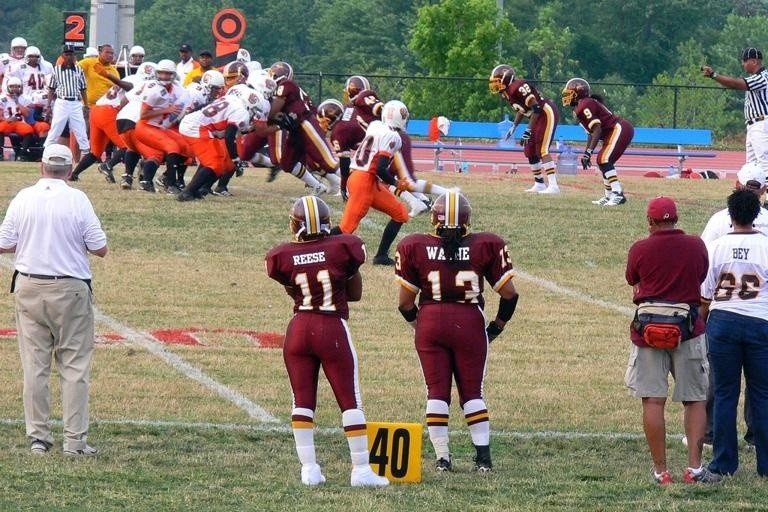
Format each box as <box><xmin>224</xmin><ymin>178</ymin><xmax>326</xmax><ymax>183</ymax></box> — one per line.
<box><xmin>31</xmin><ymin>441</ymin><xmax>52</xmax><ymax>455</ymax></box>
<box><xmin>592</xmin><ymin>192</ymin><xmax>627</xmax><ymax>207</ymax></box>
<box><xmin>312</xmin><ymin>177</ymin><xmax>341</xmax><ymax>194</ymax></box>
<box><xmin>475</xmin><ymin>463</ymin><xmax>492</xmax><ymax>474</ymax></box>
<box><xmin>300</xmin><ymin>466</ymin><xmax>326</xmax><ymax>484</ymax></box>
<box><xmin>683</xmin><ymin>464</ymin><xmax>704</xmax><ymax>482</ymax></box>
<box><xmin>63</xmin><ymin>443</ymin><xmax>99</xmax><ymax>456</ymax></box>
<box><xmin>651</xmin><ymin>469</ymin><xmax>675</xmax><ymax>485</ymax></box>
<box><xmin>373</xmin><ymin>254</ymin><xmax>397</xmax><ymax>265</ymax></box>
<box><xmin>525</xmin><ymin>183</ymin><xmax>561</xmax><ymax>196</ymax></box>
<box><xmin>350</xmin><ymin>466</ymin><xmax>392</xmax><ymax>488</ymax></box>
<box><xmin>409</xmin><ymin>198</ymin><xmax>430</xmax><ymax>217</ymax></box>
<box><xmin>436</xmin><ymin>458</ymin><xmax>453</xmax><ymax>474</ymax></box>
<box><xmin>68</xmin><ymin>165</ymin><xmax>280</xmax><ymax>202</ymax></box>
<box><xmin>681</xmin><ymin>436</ymin><xmax>713</xmax><ymax>449</ymax></box>
<box><xmin>693</xmin><ymin>468</ymin><xmax>730</xmax><ymax>486</ymax></box>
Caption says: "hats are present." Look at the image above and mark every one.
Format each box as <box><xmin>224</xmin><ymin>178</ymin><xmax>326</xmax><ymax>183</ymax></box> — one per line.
<box><xmin>62</xmin><ymin>44</ymin><xmax>75</xmax><ymax>53</ymax></box>
<box><xmin>736</xmin><ymin>162</ymin><xmax>768</xmax><ymax>189</ymax></box>
<box><xmin>180</xmin><ymin>44</ymin><xmax>193</xmax><ymax>53</ymax></box>
<box><xmin>198</xmin><ymin>49</ymin><xmax>214</xmax><ymax>57</ymax></box>
<box><xmin>739</xmin><ymin>47</ymin><xmax>764</xmax><ymax>60</ymax></box>
<box><xmin>646</xmin><ymin>197</ymin><xmax>677</xmax><ymax>222</ymax></box>
<box><xmin>41</xmin><ymin>144</ymin><xmax>74</xmax><ymax>166</ymax></box>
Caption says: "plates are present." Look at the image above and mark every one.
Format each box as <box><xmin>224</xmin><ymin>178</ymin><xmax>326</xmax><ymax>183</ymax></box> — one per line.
<box><xmin>555</xmin><ymin>136</ymin><xmax>578</xmax><ymax>174</ymax></box>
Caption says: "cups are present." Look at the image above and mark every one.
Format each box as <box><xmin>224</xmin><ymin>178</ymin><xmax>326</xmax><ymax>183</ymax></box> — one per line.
<box><xmin>492</xmin><ymin>164</ymin><xmax>499</xmax><ymax>174</ymax></box>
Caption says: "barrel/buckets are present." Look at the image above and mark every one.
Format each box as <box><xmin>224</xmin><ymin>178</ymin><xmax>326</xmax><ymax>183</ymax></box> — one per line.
<box><xmin>557</xmin><ymin>145</ymin><xmax>577</xmax><ymax>175</ymax></box>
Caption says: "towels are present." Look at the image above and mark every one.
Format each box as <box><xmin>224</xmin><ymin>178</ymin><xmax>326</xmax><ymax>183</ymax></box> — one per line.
<box><xmin>437</xmin><ymin>116</ymin><xmax>450</xmax><ymax>136</ymax></box>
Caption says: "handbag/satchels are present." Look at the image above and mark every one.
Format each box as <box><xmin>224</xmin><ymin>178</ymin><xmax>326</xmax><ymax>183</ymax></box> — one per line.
<box><xmin>633</xmin><ymin>299</ymin><xmax>699</xmax><ymax>352</ymax></box>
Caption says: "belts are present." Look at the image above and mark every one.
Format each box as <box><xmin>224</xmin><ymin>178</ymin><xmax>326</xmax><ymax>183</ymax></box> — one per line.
<box><xmin>19</xmin><ymin>270</ymin><xmax>75</xmax><ymax>280</ymax></box>
<box><xmin>58</xmin><ymin>95</ymin><xmax>82</xmax><ymax>102</ymax></box>
<box><xmin>745</xmin><ymin>114</ymin><xmax>768</xmax><ymax>126</ymax></box>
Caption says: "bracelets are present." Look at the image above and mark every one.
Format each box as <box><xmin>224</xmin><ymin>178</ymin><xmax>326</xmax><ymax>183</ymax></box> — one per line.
<box><xmin>712</xmin><ymin>72</ymin><xmax>717</xmax><ymax>79</ymax></box>
<box><xmin>710</xmin><ymin>72</ymin><xmax>714</xmax><ymax>77</ymax></box>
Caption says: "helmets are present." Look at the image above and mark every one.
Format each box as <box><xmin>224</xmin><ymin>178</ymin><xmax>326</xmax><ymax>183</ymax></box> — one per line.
<box><xmin>381</xmin><ymin>99</ymin><xmax>409</xmax><ymax>129</ymax></box>
<box><xmin>24</xmin><ymin>45</ymin><xmax>41</xmax><ymax>64</ymax></box>
<box><xmin>7</xmin><ymin>77</ymin><xmax>23</xmax><ymax>95</ymax></box>
<box><xmin>10</xmin><ymin>36</ymin><xmax>26</xmax><ymax>58</ymax></box>
<box><xmin>317</xmin><ymin>98</ymin><xmax>344</xmax><ymax>130</ymax></box>
<box><xmin>346</xmin><ymin>76</ymin><xmax>370</xmax><ymax>101</ymax></box>
<box><xmin>563</xmin><ymin>77</ymin><xmax>593</xmax><ymax>106</ymax></box>
<box><xmin>288</xmin><ymin>196</ymin><xmax>333</xmax><ymax>237</ymax></box>
<box><xmin>139</xmin><ymin>62</ymin><xmax>295</xmax><ymax>91</ymax></box>
<box><xmin>128</xmin><ymin>46</ymin><xmax>145</xmax><ymax>58</ymax></box>
<box><xmin>431</xmin><ymin>192</ymin><xmax>472</xmax><ymax>235</ymax></box>
<box><xmin>489</xmin><ymin>65</ymin><xmax>516</xmax><ymax>92</ymax></box>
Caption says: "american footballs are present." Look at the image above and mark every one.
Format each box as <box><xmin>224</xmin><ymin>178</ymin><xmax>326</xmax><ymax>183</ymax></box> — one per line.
<box><xmin>168</xmin><ymin>99</ymin><xmax>185</xmax><ymax>123</ymax></box>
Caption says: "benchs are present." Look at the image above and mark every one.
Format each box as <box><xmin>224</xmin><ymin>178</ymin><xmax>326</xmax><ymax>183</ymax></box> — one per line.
<box><xmin>401</xmin><ymin>116</ymin><xmax>718</xmax><ymax>179</ymax></box>
<box><xmin>0</xmin><ymin>107</ymin><xmax>48</xmax><ymax>152</ymax></box>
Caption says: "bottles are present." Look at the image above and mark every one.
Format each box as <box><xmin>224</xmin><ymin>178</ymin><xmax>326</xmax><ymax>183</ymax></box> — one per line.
<box><xmin>455</xmin><ymin>159</ymin><xmax>468</xmax><ymax>173</ymax></box>
<box><xmin>506</xmin><ymin>163</ymin><xmax>517</xmax><ymax>174</ymax></box>
<box><xmin>455</xmin><ymin>138</ymin><xmax>462</xmax><ymax>146</ymax></box>
<box><xmin>668</xmin><ymin>165</ymin><xmax>674</xmax><ymax>175</ymax></box>
<box><xmin>439</xmin><ymin>161</ymin><xmax>444</xmax><ymax>171</ymax></box>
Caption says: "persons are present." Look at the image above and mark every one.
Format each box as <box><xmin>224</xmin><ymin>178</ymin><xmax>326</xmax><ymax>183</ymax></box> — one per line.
<box><xmin>264</xmin><ymin>195</ymin><xmax>389</xmax><ymax>487</ymax></box>
<box><xmin>682</xmin><ymin>162</ymin><xmax>768</xmax><ymax>450</ymax></box>
<box><xmin>562</xmin><ymin>78</ymin><xmax>634</xmax><ymax>206</ymax></box>
<box><xmin>0</xmin><ymin>142</ymin><xmax>108</xmax><ymax>457</ymax></box>
<box><xmin>488</xmin><ymin>64</ymin><xmax>561</xmax><ymax>194</ymax></box>
<box><xmin>699</xmin><ymin>187</ymin><xmax>768</xmax><ymax>478</ymax></box>
<box><xmin>704</xmin><ymin>47</ymin><xmax>768</xmax><ymax>186</ymax></box>
<box><xmin>625</xmin><ymin>196</ymin><xmax>722</xmax><ymax>484</ymax></box>
<box><xmin>395</xmin><ymin>191</ymin><xmax>519</xmax><ymax>474</ymax></box>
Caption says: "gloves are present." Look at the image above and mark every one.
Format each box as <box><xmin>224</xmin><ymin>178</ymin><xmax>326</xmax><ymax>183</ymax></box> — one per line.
<box><xmin>504</xmin><ymin>126</ymin><xmax>516</xmax><ymax>143</ymax></box>
<box><xmin>580</xmin><ymin>150</ymin><xmax>595</xmax><ymax>170</ymax></box>
<box><xmin>279</xmin><ymin>111</ymin><xmax>297</xmax><ymax>132</ymax></box>
<box><xmin>395</xmin><ymin>179</ymin><xmax>410</xmax><ymax>192</ymax></box>
<box><xmin>340</xmin><ymin>186</ymin><xmax>349</xmax><ymax>203</ymax></box>
<box><xmin>518</xmin><ymin>127</ymin><xmax>533</xmax><ymax>147</ymax></box>
